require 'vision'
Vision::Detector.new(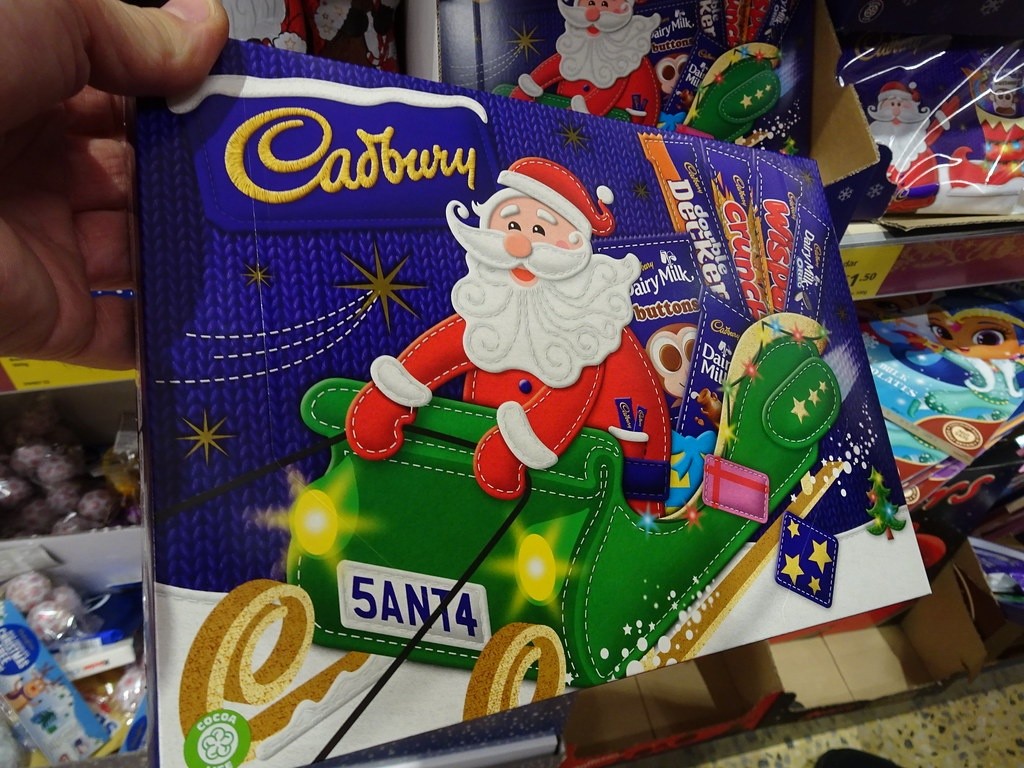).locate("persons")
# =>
[1,2,232,374]
